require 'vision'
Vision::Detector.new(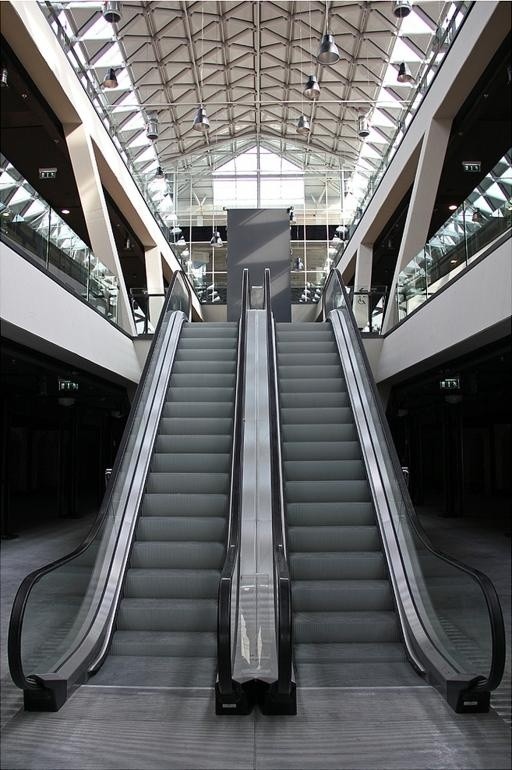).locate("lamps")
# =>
[104,2,212,139]
[210,211,222,246]
[295,242,303,270]
[290,208,297,223]
[296,0,417,138]
